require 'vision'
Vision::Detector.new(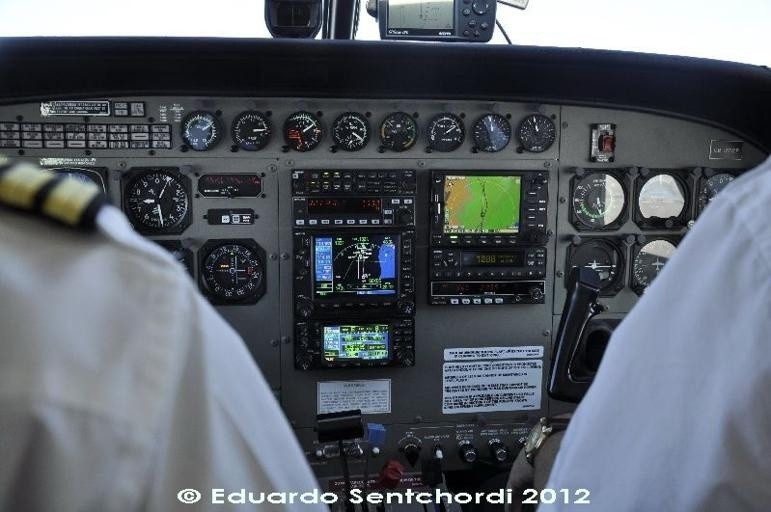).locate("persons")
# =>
[538,158,769,512]
[0,155,332,512]
